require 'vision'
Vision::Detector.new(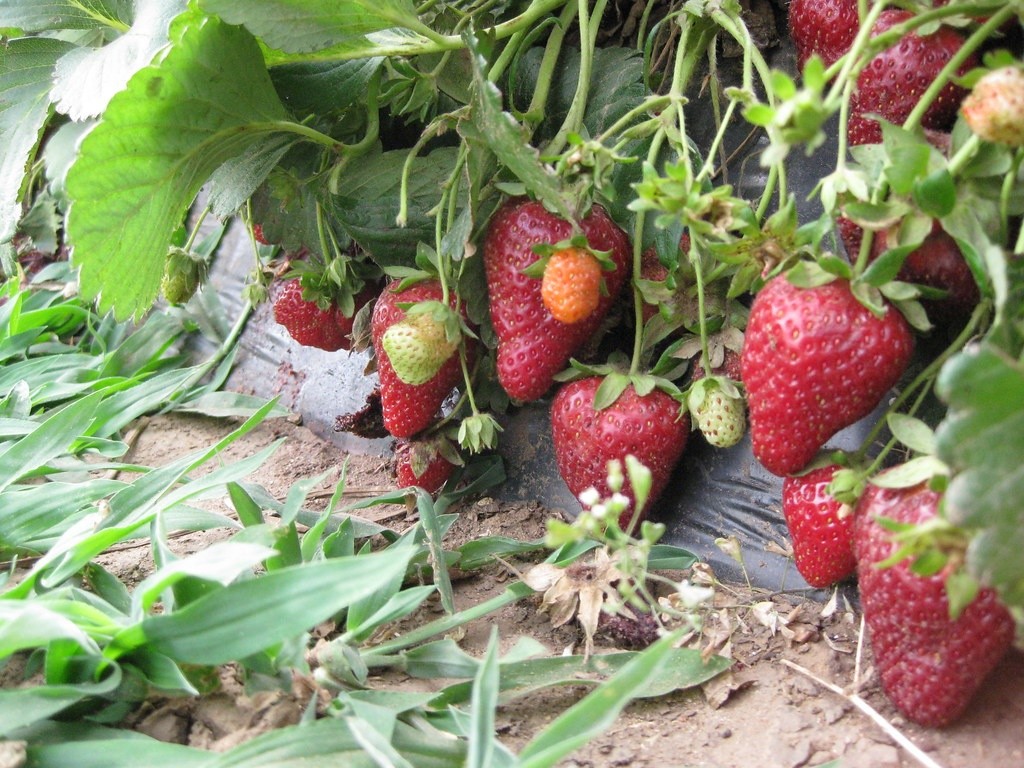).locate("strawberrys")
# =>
[252,1,1024,731]
